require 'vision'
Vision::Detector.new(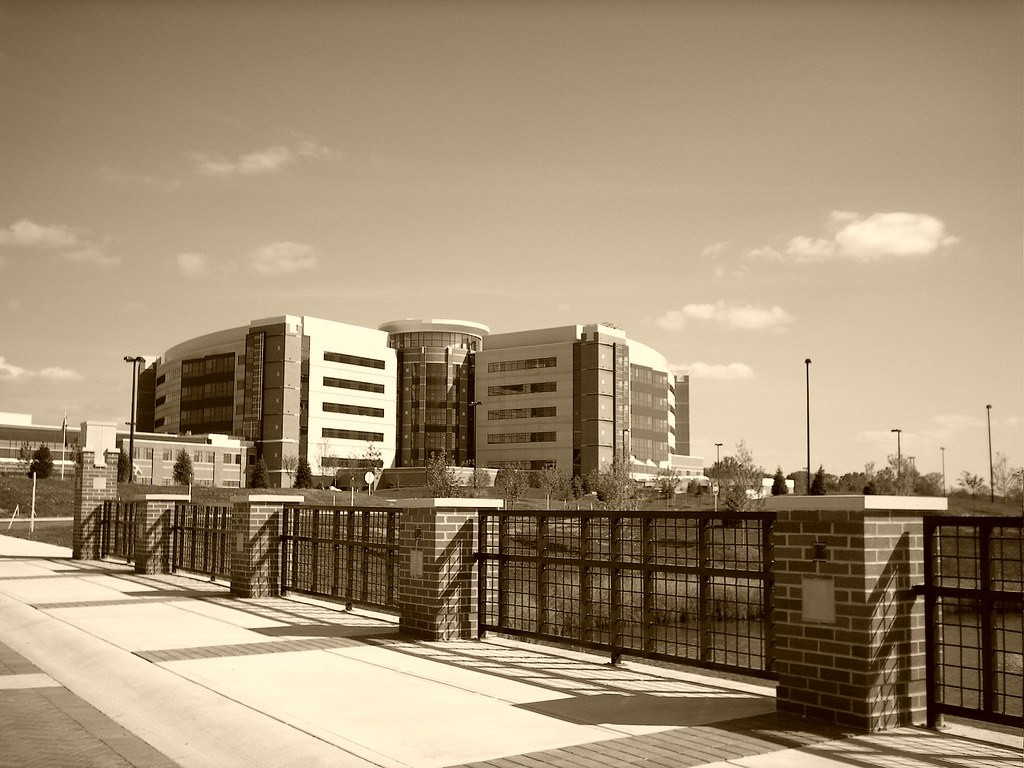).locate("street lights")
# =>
[987,404,996,503]
[892,428,901,477]
[714,443,723,467]
[469,402,484,489]
[804,358,813,495]
[941,447,947,497]
[622,428,628,469]
[123,355,145,484]
